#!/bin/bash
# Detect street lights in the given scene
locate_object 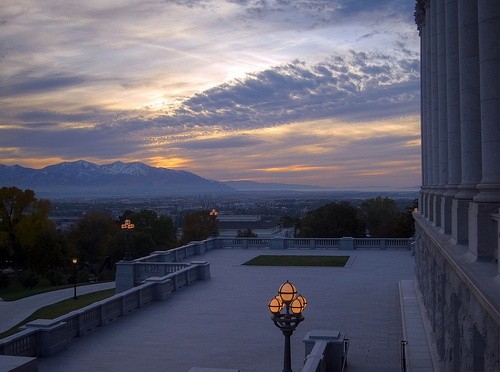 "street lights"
[268,280,306,372]
[210,209,218,238]
[121,219,136,262]
[72,257,78,300]
